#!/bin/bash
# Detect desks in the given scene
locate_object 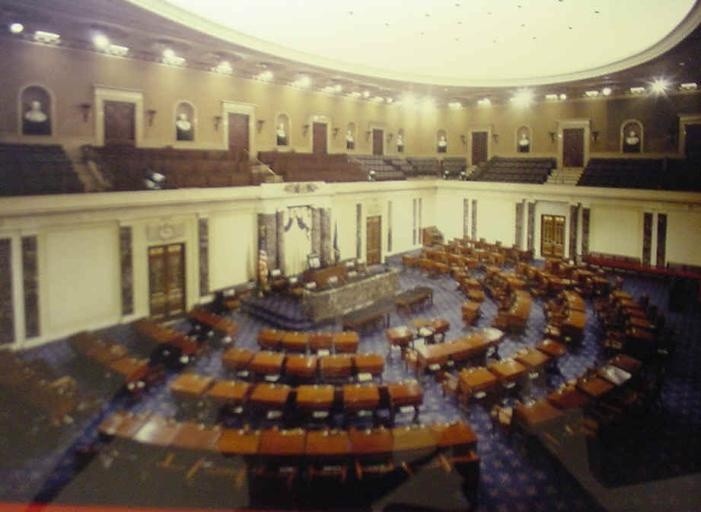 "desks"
[135,318,201,363]
[257,328,359,354]
[343,306,390,338]
[453,366,497,414]
[170,372,215,411]
[11,361,81,417]
[319,355,353,381]
[609,353,643,376]
[577,375,613,399]
[415,325,508,369]
[516,347,549,374]
[536,337,566,368]
[306,424,477,480]
[293,383,337,416]
[597,365,633,389]
[401,236,609,275]
[383,319,450,358]
[249,382,293,413]
[285,355,318,380]
[250,351,284,379]
[486,356,526,400]
[221,346,251,378]
[460,275,654,344]
[93,411,305,478]
[394,286,433,319]
[513,397,561,430]
[73,331,151,394]
[210,380,253,414]
[386,382,425,421]
[354,355,384,381]
[189,305,236,336]
[547,384,585,413]
[341,383,381,413]
